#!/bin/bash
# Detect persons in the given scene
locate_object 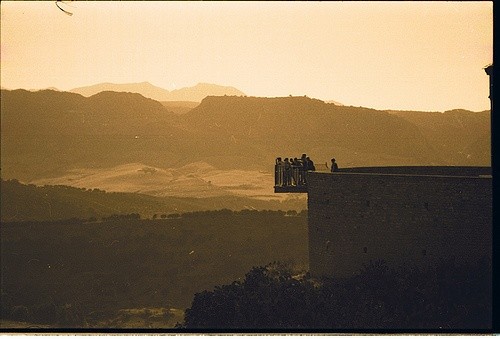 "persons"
[330,159,337,172]
[275,153,315,186]
